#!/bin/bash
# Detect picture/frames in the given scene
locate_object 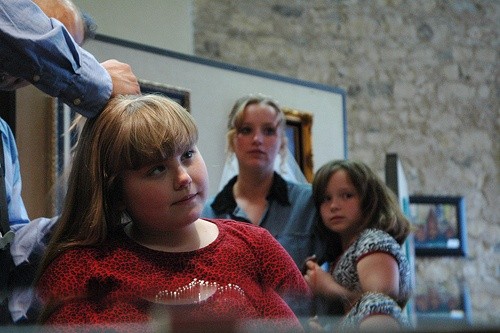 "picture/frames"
[283,108,315,186]
[405,195,468,258]
[47,68,191,217]
